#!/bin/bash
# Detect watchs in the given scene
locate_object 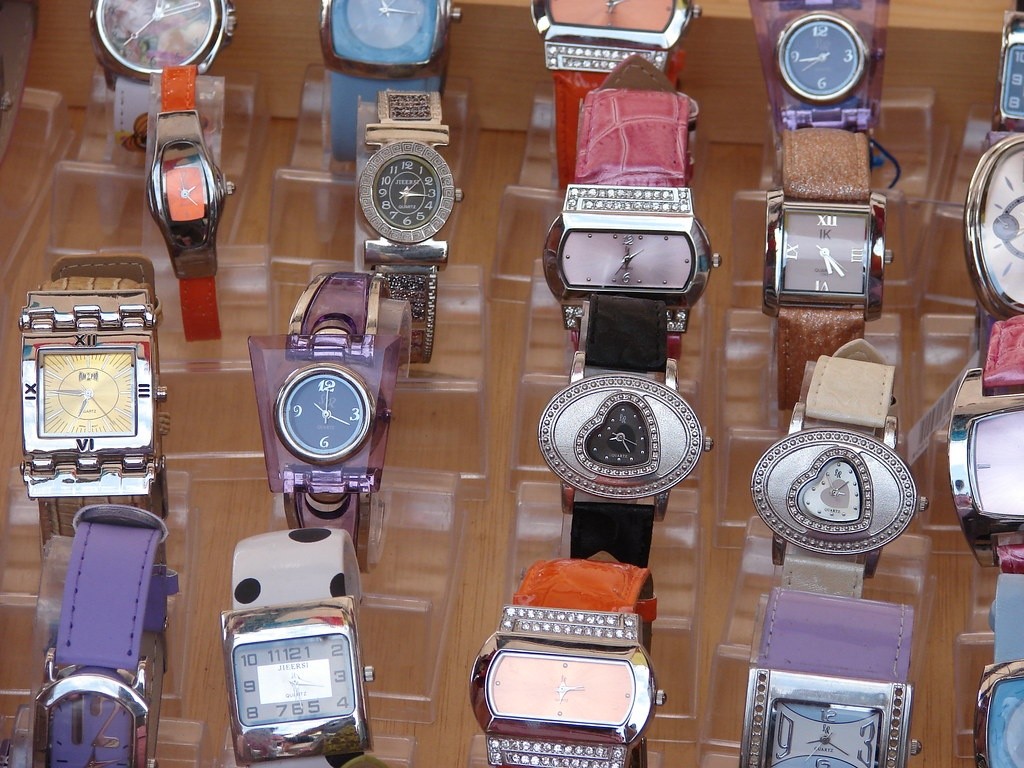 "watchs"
[1,0,1023,767]
